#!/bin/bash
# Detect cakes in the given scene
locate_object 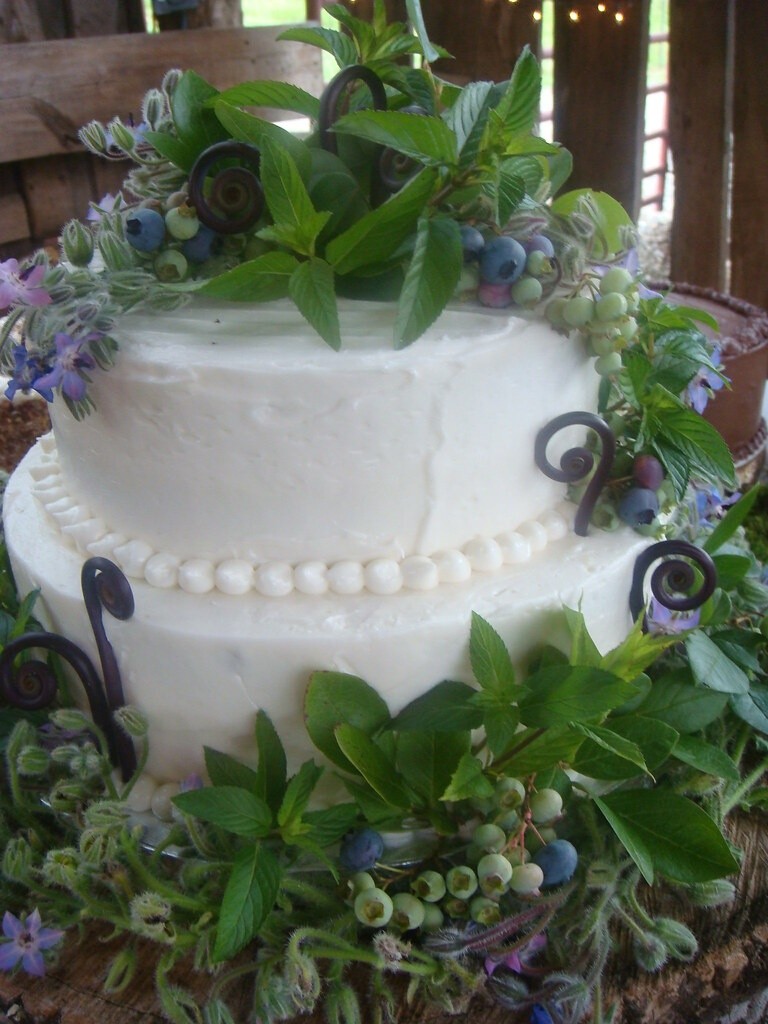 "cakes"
[0,0,767,824]
